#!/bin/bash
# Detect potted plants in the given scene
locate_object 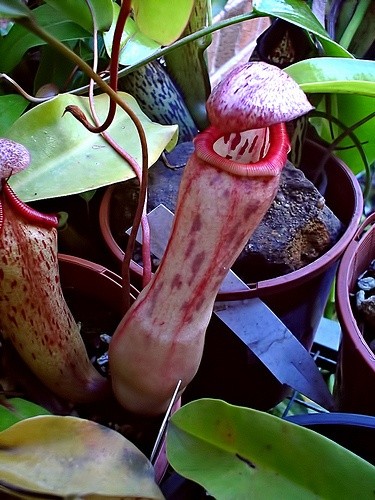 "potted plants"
[0,0,375,500]
